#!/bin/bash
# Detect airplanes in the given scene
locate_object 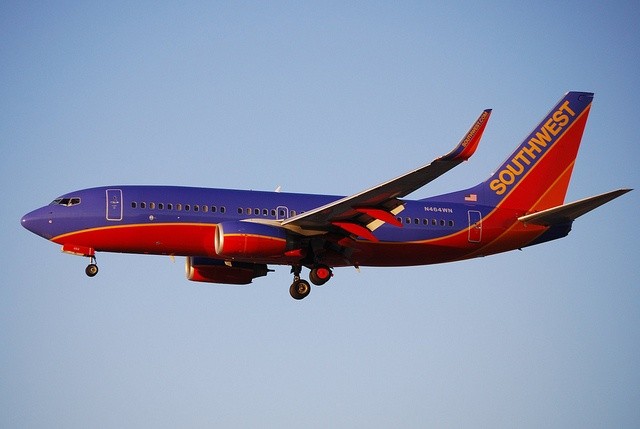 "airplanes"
[21,90,635,300]
